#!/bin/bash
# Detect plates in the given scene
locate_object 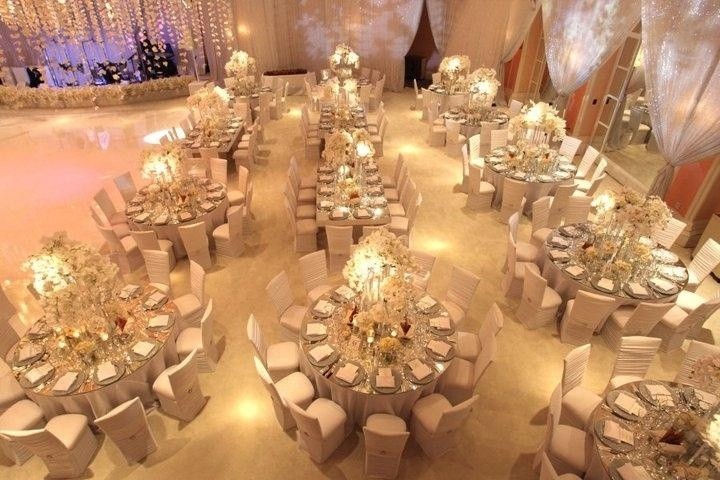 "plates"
[484,141,577,184]
[592,377,720,479]
[428,81,480,96]
[124,175,226,224]
[442,103,510,128]
[318,73,370,87]
[13,283,177,398]
[226,83,272,100]
[300,284,458,395]
[319,104,367,132]
[181,112,243,152]
[546,223,688,301]
[315,156,387,222]
